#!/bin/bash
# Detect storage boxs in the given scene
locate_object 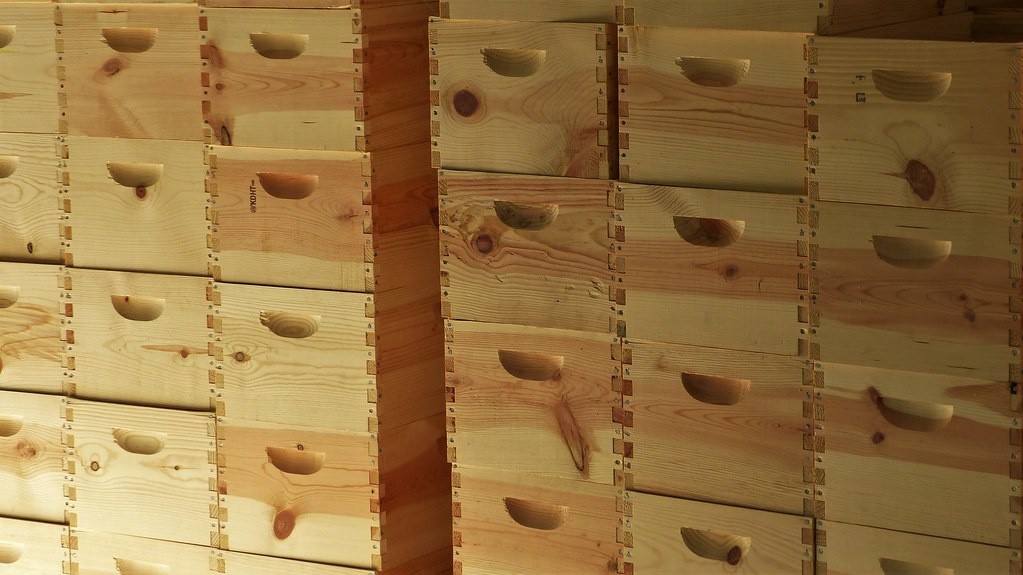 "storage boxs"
[0,1,1023,575]
